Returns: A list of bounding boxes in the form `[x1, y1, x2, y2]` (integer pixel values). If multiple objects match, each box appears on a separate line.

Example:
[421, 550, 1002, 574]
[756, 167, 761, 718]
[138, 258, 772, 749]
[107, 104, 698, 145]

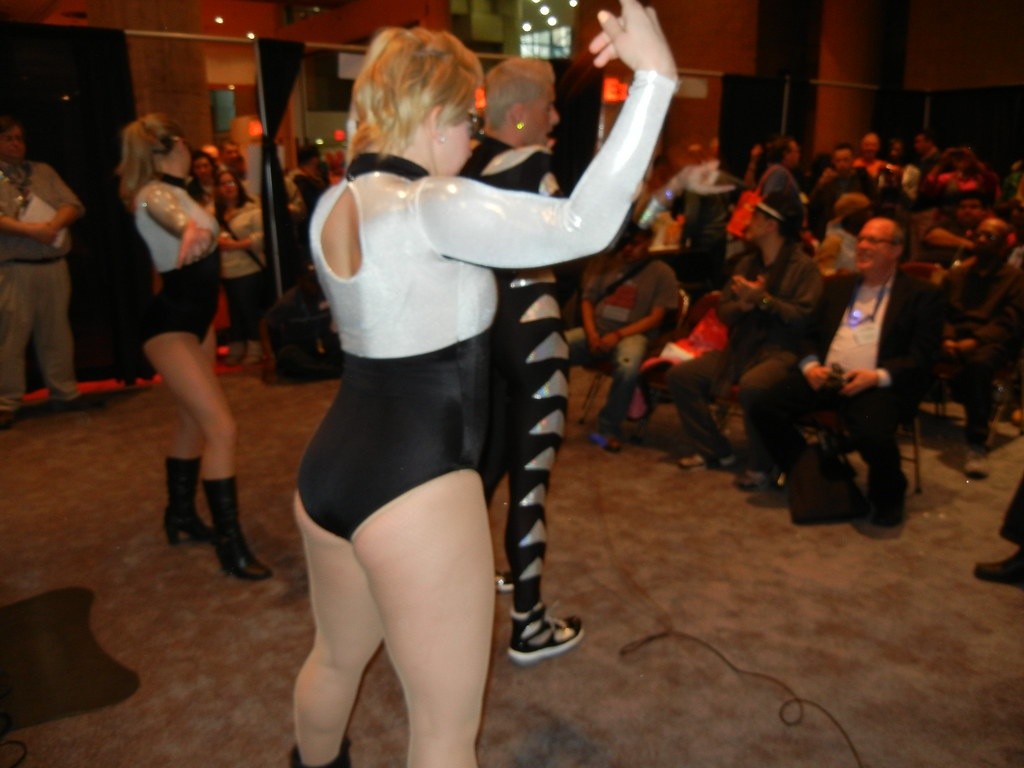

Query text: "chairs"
[565, 213, 1024, 495]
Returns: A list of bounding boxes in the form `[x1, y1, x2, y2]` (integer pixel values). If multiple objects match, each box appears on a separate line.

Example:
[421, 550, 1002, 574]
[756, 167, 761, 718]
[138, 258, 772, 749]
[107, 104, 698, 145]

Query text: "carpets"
[0, 585, 142, 742]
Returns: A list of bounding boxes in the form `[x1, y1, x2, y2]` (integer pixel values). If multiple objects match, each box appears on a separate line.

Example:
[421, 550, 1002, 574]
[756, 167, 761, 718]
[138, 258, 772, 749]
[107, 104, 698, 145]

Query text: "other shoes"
[680, 455, 713, 468]
[225, 341, 265, 365]
[965, 449, 994, 476]
[597, 433, 621, 454]
[872, 508, 903, 528]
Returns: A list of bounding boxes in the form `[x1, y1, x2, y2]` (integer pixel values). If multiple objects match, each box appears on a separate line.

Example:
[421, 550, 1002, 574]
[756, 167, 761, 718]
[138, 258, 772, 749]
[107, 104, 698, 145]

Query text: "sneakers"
[508, 615, 585, 665]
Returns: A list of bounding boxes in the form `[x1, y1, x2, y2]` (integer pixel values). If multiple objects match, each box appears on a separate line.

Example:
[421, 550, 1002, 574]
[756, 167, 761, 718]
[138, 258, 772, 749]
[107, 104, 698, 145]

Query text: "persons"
[463, 56, 584, 663]
[285, 144, 340, 356]
[567, 132, 1024, 586]
[191, 142, 263, 364]
[287, 0, 679, 768]
[120, 112, 273, 581]
[0, 119, 99, 427]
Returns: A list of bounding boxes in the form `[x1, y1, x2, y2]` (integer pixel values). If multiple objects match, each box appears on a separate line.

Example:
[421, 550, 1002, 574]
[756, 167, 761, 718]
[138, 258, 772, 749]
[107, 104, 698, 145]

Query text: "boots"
[163, 456, 212, 546]
[203, 477, 272, 580]
[493, 571, 514, 592]
[291, 738, 352, 768]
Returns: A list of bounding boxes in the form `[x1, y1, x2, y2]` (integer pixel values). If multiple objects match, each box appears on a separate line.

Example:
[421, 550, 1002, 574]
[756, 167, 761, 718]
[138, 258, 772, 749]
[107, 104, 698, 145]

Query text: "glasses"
[857, 234, 896, 247]
[465, 113, 486, 135]
[1, 135, 24, 142]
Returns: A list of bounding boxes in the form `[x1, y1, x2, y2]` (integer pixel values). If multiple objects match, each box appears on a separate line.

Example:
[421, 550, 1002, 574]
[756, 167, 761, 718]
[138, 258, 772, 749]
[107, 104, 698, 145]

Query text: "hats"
[750, 189, 805, 230]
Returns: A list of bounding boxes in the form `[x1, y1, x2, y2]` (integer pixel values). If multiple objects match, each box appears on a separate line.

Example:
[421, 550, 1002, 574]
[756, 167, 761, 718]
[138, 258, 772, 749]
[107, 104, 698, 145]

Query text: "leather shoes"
[974, 549, 1024, 587]
[2, 392, 104, 428]
